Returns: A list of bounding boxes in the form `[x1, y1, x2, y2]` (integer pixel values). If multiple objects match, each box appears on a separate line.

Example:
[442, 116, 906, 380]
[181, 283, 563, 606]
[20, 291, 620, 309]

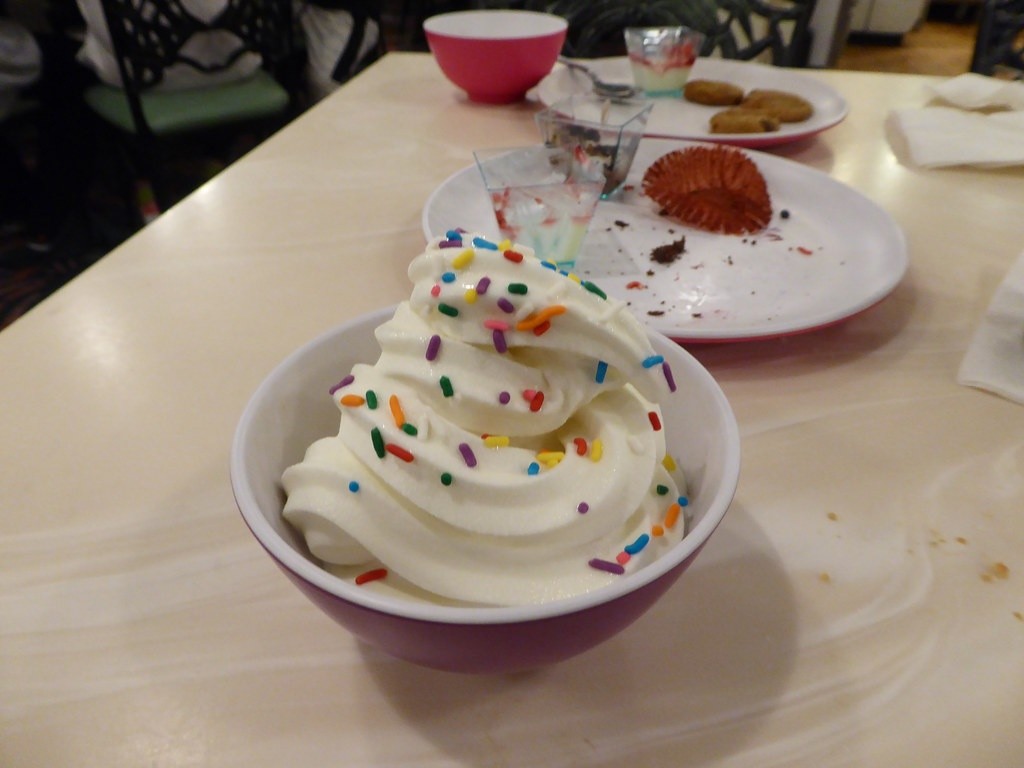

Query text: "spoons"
[558, 54, 635, 96]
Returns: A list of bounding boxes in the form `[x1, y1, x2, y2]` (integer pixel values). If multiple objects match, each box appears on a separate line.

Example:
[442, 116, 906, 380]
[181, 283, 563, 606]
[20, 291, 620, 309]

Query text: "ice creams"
[279, 227, 687, 609]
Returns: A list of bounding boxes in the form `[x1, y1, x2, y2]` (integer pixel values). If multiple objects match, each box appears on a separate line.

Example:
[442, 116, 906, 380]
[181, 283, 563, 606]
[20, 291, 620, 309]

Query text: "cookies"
[739, 89, 814, 123]
[683, 80, 743, 106]
[710, 108, 780, 133]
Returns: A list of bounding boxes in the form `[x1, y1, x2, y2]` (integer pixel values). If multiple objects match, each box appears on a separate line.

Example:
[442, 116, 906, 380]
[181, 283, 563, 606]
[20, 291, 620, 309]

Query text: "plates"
[422, 137, 911, 343]
[525, 54, 852, 150]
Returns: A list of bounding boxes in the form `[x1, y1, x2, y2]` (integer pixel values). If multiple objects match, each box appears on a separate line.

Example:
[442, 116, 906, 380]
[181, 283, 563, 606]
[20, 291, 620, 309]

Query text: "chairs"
[45, 0, 819, 218]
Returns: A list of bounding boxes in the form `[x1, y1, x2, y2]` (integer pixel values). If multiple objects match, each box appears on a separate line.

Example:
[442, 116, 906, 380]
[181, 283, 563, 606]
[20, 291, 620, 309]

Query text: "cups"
[625, 24, 708, 97]
[536, 92, 654, 199]
[472, 144, 611, 270]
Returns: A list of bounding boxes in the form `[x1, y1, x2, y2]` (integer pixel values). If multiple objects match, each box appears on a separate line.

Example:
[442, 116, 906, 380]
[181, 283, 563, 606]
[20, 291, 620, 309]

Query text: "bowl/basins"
[422, 10, 570, 105]
[230, 305, 744, 674]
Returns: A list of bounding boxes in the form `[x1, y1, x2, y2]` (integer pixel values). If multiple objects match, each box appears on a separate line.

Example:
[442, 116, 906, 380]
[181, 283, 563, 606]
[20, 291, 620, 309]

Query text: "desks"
[0, 51, 1024, 768]
